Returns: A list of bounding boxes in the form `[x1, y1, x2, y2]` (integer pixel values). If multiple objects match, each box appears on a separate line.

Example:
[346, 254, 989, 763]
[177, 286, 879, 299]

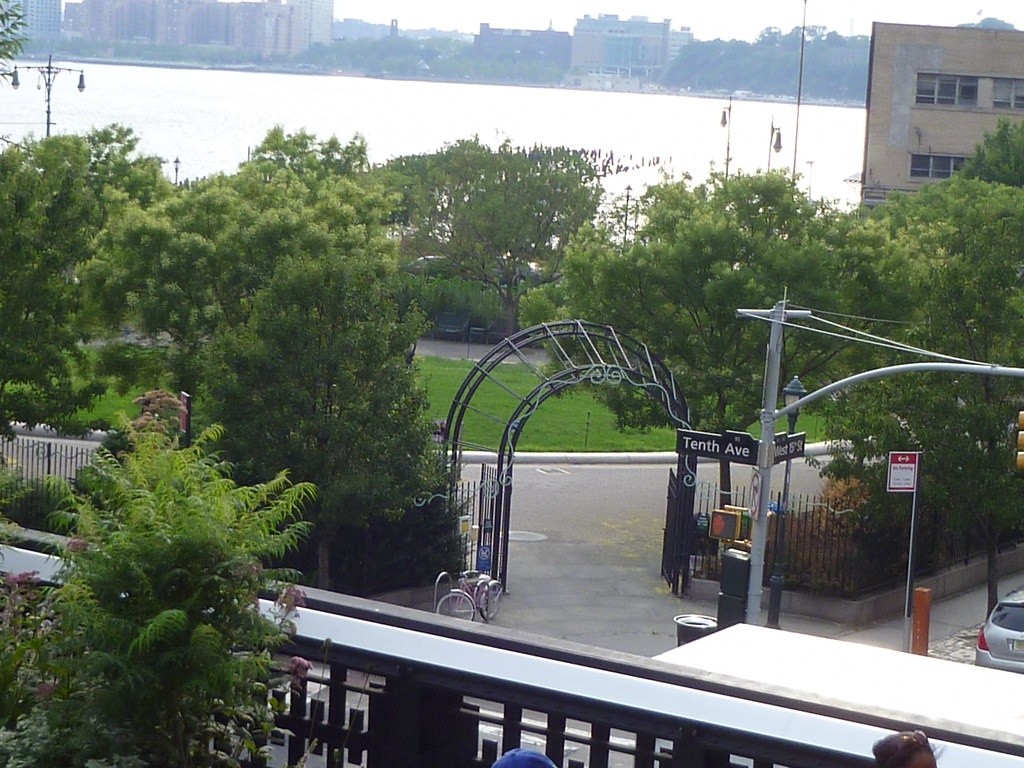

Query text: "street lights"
[764, 376, 810, 638]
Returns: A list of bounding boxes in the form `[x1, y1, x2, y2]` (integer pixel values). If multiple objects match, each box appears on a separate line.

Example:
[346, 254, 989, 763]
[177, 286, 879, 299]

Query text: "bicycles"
[436, 570, 503, 622]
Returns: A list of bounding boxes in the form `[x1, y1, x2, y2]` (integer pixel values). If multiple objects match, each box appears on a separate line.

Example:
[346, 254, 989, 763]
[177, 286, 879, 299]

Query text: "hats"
[491, 748, 557, 768]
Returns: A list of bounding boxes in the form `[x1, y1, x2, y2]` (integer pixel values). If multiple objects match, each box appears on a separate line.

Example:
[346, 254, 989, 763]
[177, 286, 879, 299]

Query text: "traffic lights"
[709, 510, 742, 542]
[1016, 411, 1024, 470]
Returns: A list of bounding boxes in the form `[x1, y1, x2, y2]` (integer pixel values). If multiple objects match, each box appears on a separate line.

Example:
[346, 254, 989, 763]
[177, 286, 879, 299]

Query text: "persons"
[871, 730, 938, 768]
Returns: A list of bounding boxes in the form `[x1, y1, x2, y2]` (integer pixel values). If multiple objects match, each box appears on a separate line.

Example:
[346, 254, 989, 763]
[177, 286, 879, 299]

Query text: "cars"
[973, 590, 1024, 674]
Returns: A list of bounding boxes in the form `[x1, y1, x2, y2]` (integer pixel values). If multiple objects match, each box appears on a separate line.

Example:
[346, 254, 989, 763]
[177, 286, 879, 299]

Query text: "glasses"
[884, 729, 927, 768]
